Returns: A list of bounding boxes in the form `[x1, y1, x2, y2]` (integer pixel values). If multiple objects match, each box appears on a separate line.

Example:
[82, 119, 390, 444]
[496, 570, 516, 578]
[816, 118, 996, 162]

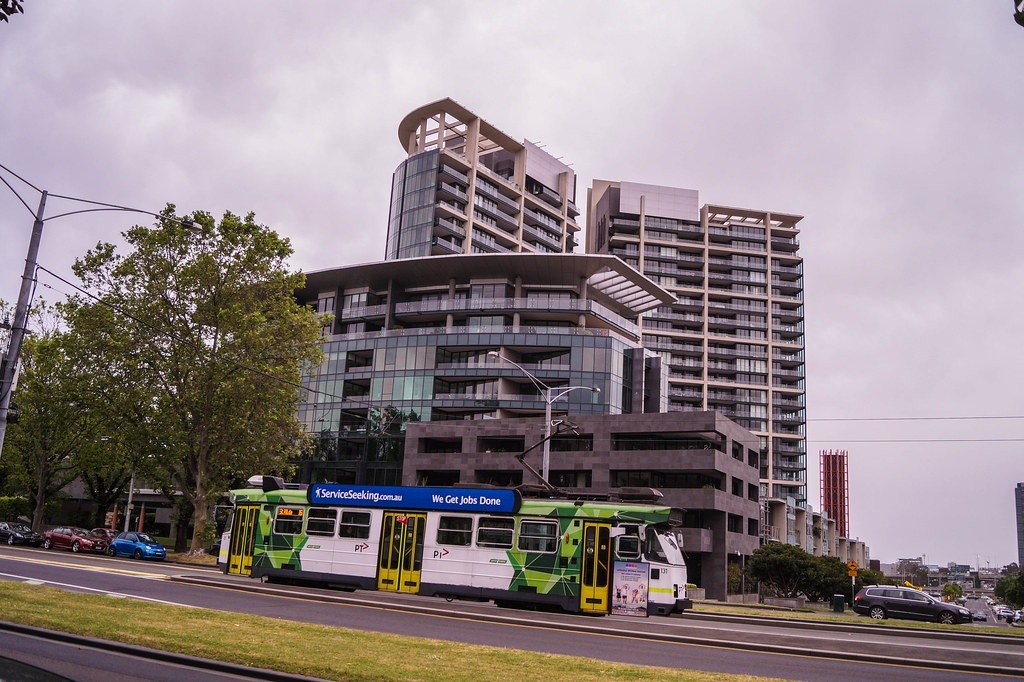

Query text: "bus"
[213, 475, 692, 618]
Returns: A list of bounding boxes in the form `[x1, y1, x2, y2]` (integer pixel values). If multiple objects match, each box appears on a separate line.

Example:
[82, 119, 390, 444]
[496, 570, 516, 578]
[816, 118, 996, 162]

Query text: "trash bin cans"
[833, 594, 845, 613]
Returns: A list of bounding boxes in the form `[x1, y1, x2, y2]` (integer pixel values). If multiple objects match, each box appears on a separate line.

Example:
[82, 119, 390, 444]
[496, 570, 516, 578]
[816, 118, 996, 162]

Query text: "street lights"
[0, 165, 203, 456]
[488, 351, 601, 487]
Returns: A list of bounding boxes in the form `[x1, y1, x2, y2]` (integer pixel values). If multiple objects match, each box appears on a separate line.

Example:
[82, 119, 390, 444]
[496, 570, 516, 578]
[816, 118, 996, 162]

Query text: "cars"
[91, 528, 122, 544]
[0, 522, 43, 547]
[43, 526, 107, 553]
[987, 598, 995, 605]
[108, 531, 167, 560]
[992, 605, 1024, 620]
[972, 611, 988, 622]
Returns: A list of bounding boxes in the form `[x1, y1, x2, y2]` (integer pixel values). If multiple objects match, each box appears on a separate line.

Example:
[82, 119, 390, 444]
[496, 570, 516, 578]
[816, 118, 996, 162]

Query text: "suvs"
[853, 584, 972, 625]
